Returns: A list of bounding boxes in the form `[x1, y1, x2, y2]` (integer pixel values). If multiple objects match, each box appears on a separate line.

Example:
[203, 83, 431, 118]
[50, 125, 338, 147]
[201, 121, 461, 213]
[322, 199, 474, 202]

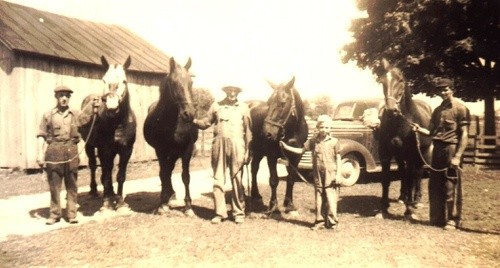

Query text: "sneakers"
[46, 217, 60, 225]
[235, 215, 245, 223]
[210, 216, 222, 224]
[68, 219, 78, 223]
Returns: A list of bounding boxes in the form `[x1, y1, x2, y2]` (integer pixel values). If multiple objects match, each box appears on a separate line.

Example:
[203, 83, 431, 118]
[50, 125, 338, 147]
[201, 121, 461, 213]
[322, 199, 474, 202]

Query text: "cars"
[276, 96, 436, 187]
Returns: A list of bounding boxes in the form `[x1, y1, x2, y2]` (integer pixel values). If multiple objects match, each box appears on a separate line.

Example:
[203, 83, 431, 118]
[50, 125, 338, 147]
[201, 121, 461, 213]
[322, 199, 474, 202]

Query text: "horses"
[78, 53, 138, 214]
[368, 57, 432, 220]
[142, 56, 196, 218]
[240, 76, 309, 220]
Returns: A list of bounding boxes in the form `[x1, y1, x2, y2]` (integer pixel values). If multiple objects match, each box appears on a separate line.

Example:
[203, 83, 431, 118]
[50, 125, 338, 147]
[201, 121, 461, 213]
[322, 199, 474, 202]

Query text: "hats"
[434, 78, 452, 88]
[222, 84, 242, 93]
[54, 86, 73, 93]
[316, 115, 334, 129]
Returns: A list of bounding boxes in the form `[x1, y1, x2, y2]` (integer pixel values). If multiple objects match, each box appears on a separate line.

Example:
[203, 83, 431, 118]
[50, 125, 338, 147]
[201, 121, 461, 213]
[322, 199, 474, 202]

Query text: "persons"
[408, 78, 470, 230]
[278, 114, 342, 230]
[35, 86, 81, 224]
[190, 81, 250, 224]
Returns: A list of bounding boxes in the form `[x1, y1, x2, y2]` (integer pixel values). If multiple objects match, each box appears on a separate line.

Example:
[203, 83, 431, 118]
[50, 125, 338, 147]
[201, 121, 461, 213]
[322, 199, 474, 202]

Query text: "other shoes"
[312, 222, 336, 232]
[430, 220, 458, 231]
